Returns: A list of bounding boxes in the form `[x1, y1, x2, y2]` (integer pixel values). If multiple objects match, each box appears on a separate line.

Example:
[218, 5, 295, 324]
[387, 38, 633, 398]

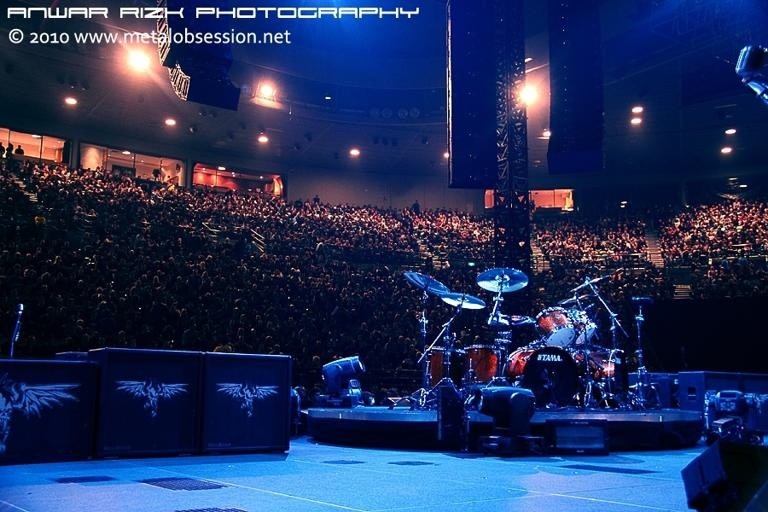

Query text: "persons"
[1, 139, 766, 405]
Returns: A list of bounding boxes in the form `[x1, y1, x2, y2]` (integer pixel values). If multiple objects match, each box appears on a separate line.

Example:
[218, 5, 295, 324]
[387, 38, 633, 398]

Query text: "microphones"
[17, 303, 24, 311]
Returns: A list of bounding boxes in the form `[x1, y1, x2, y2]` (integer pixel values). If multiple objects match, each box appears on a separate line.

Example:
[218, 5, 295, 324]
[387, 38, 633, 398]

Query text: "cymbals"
[401, 271, 451, 296]
[476, 267, 528, 292]
[557, 295, 591, 305]
[571, 275, 608, 292]
[440, 293, 486, 310]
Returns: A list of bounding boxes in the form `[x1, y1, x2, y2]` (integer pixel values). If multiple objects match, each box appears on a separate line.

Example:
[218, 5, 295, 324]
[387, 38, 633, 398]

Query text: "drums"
[505, 345, 578, 406]
[495, 331, 512, 344]
[462, 346, 508, 384]
[575, 352, 617, 386]
[567, 310, 597, 345]
[536, 307, 575, 349]
[428, 346, 466, 388]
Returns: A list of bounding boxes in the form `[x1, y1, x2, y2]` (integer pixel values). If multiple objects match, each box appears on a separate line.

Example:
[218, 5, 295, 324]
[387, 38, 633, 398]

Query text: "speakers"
[201, 351, 292, 452]
[0, 358, 97, 465]
[628, 371, 767, 433]
[680, 438, 767, 512]
[88, 349, 198, 458]
[156, 0, 240, 112]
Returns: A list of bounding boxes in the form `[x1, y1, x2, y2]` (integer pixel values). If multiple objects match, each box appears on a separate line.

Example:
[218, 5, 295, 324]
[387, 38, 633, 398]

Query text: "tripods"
[386, 321, 663, 413]
